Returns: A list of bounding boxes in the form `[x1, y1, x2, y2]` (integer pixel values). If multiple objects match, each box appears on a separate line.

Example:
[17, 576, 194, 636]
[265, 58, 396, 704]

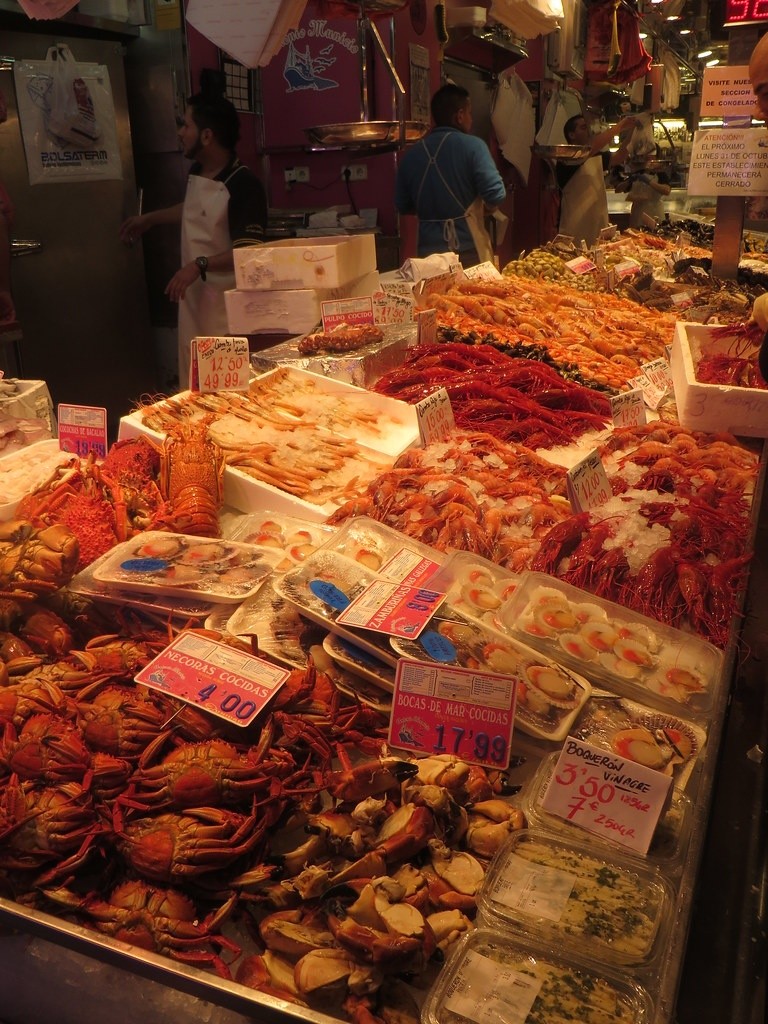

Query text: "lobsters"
[18, 414, 225, 569]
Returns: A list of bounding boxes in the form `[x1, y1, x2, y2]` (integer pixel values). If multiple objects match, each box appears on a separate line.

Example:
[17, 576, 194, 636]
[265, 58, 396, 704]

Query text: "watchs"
[195, 256, 208, 282]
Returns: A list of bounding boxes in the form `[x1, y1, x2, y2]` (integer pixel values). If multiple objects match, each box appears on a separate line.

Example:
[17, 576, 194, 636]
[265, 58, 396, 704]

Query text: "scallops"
[64, 506, 726, 800]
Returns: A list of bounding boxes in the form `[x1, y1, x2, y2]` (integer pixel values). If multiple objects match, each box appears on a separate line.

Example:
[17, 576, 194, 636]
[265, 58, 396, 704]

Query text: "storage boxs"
[669, 321, 768, 438]
[0, 379, 55, 420]
[248, 364, 421, 457]
[232, 233, 377, 292]
[117, 385, 396, 523]
[225, 270, 380, 334]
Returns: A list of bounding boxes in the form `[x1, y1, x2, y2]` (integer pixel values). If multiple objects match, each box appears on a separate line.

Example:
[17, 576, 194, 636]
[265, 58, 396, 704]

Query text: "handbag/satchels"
[13, 43, 126, 186]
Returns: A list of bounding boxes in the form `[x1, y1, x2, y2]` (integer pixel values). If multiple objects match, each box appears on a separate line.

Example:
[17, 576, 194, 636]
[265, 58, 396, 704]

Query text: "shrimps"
[128, 374, 402, 510]
[326, 278, 762, 651]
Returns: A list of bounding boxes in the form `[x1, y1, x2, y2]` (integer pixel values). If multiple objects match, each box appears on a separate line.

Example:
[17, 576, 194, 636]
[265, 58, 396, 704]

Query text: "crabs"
[0, 516, 391, 982]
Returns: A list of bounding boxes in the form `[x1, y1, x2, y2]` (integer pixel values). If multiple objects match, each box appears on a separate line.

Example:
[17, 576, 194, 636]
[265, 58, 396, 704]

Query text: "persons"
[394, 85, 507, 271]
[120, 91, 266, 395]
[615, 143, 673, 229]
[552, 112, 640, 246]
[746, 28, 768, 385]
